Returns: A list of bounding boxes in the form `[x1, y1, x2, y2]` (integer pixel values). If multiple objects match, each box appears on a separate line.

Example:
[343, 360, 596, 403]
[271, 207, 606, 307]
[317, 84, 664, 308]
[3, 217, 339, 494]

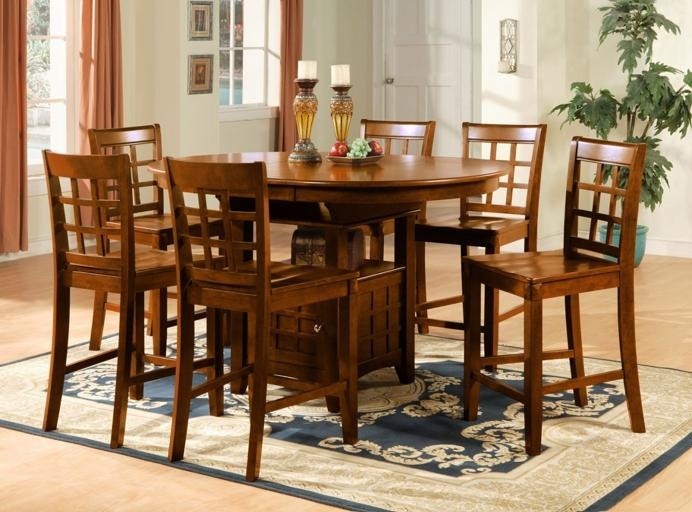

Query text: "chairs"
[298, 118, 436, 334]
[414, 122, 547, 373]
[462, 135, 647, 455]
[41, 149, 225, 449]
[163, 156, 360, 482]
[88, 123, 230, 365]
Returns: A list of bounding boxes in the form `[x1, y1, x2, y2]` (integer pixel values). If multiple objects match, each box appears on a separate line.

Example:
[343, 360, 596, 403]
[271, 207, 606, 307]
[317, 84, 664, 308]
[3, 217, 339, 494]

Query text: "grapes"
[346, 138, 372, 158]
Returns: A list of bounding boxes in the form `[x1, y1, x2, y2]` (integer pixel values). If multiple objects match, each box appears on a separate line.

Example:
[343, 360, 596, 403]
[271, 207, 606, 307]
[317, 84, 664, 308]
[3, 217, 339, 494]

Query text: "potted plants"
[547, 1, 692, 267]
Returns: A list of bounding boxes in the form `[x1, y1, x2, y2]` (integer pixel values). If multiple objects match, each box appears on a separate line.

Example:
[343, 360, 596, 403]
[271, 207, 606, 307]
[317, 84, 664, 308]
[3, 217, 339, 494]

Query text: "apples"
[368, 141, 383, 156]
[330, 142, 348, 157]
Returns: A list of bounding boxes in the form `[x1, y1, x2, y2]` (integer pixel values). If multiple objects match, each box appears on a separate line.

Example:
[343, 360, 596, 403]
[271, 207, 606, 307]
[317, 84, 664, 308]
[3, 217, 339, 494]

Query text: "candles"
[297, 60, 317, 79]
[331, 64, 351, 84]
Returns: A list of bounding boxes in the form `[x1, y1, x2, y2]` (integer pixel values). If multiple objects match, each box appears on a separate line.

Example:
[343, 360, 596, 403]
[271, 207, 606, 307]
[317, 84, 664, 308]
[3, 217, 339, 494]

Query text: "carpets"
[1, 306, 692, 511]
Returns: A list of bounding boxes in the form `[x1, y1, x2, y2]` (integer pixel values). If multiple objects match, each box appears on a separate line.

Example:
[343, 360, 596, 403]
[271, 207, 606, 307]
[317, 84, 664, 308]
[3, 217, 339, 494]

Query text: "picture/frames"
[187, 0, 213, 41]
[188, 55, 213, 94]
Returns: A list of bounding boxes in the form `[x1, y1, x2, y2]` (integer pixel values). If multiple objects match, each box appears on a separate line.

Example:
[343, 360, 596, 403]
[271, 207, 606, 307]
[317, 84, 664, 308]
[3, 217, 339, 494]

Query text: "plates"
[326, 154, 384, 167]
